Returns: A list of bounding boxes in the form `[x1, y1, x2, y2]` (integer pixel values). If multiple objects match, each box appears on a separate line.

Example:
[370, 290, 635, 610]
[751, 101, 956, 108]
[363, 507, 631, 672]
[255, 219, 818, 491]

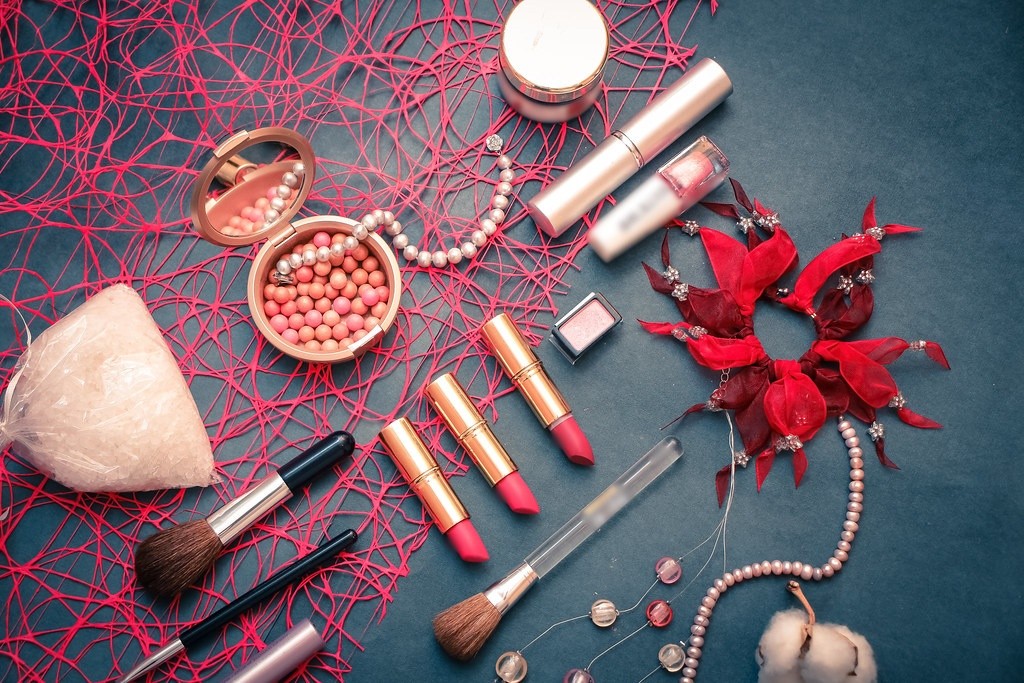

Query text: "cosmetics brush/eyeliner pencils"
[113, 528, 357, 681]
[430, 437, 685, 665]
[132, 431, 357, 607]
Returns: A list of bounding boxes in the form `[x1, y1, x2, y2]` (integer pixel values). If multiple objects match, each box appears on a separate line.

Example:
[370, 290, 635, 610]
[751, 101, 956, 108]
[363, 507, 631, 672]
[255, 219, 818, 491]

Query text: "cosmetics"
[493, 0, 611, 127]
[527, 59, 733, 238]
[377, 416, 492, 564]
[421, 369, 540, 516]
[480, 311, 596, 468]
[586, 134, 731, 263]
[550, 288, 624, 367]
[222, 618, 327, 683]
